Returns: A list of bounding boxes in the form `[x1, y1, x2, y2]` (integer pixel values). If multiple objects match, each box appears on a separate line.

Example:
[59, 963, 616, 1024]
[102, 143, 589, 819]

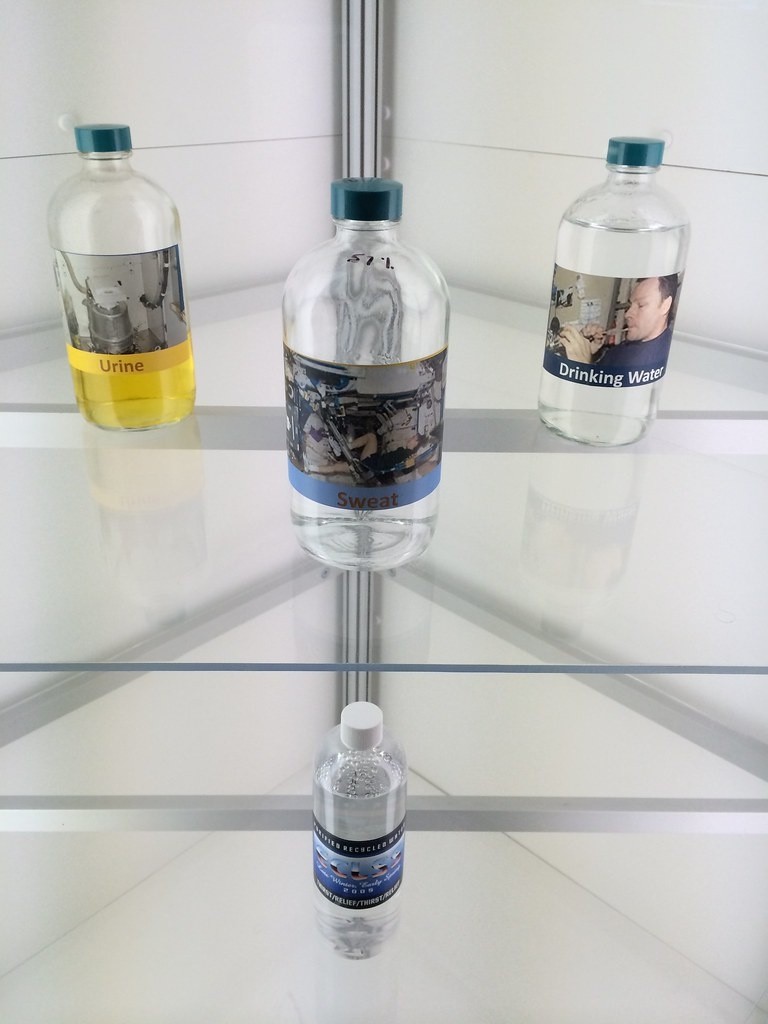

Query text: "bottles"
[47, 124, 195, 430]
[283, 177, 449, 571]
[312, 702, 406, 928]
[538, 136, 689, 447]
